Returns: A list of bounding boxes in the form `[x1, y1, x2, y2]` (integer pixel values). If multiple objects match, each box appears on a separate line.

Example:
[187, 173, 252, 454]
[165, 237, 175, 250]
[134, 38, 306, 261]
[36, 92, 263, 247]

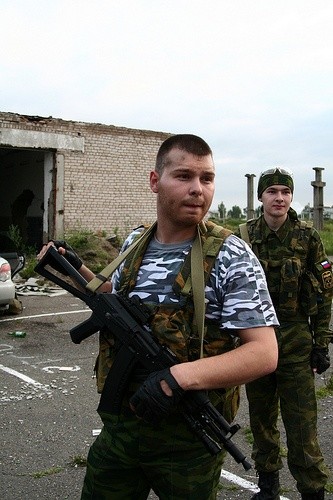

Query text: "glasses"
[261, 168, 290, 178]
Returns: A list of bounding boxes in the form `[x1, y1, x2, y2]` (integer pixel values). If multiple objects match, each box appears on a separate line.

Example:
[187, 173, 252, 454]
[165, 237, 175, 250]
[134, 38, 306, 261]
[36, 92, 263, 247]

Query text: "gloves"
[310, 343, 331, 375]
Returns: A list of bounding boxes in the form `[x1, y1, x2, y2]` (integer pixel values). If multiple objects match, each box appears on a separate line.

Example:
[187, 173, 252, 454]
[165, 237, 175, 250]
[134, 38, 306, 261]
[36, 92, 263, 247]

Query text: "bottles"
[7, 331, 26, 338]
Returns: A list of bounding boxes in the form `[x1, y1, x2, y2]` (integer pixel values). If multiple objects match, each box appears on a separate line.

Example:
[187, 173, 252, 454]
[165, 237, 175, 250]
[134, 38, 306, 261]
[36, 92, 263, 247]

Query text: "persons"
[37, 134, 281, 500]
[233, 168, 333, 500]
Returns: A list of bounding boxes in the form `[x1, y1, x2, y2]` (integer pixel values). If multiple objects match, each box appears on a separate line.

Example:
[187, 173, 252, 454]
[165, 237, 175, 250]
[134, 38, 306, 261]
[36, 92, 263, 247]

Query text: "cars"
[0, 233, 26, 306]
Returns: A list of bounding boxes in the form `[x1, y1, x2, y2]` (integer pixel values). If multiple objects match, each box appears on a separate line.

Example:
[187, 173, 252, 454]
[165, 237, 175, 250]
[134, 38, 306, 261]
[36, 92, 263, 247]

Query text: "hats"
[258, 171, 293, 196]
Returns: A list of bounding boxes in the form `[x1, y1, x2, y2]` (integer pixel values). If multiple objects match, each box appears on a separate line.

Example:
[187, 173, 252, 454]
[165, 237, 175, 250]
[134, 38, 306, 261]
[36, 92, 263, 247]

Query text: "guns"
[34, 245, 252, 473]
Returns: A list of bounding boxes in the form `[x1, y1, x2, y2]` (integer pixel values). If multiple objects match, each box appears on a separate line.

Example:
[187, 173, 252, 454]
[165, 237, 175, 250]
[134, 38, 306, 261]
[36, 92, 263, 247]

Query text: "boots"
[251, 472, 279, 500]
